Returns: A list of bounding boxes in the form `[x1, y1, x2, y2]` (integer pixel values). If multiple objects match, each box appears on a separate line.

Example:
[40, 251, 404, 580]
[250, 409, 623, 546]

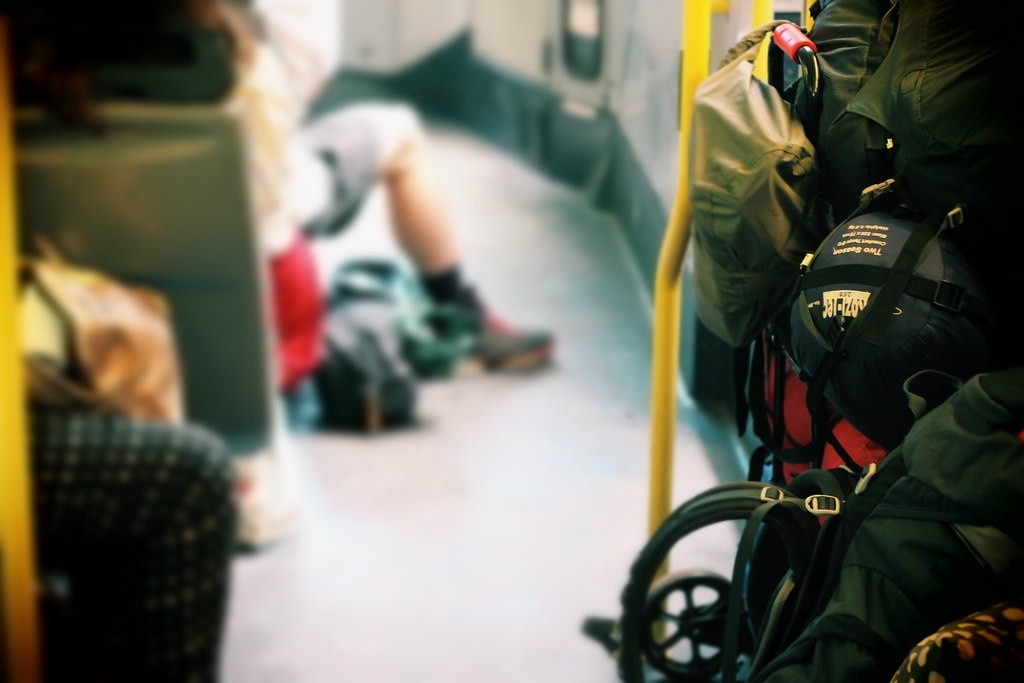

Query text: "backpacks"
[315, 297, 418, 432]
[693, 0, 1024, 683]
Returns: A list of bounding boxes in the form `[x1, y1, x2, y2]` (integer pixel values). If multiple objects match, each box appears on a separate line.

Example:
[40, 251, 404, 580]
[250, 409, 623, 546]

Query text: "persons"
[0, 1, 557, 683]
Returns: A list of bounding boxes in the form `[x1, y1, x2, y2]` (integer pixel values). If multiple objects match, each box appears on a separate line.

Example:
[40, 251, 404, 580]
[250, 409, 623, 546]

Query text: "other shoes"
[453, 283, 555, 375]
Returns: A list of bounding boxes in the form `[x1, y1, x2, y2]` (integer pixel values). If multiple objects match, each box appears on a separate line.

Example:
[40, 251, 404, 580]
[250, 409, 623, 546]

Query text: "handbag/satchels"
[330, 259, 468, 374]
